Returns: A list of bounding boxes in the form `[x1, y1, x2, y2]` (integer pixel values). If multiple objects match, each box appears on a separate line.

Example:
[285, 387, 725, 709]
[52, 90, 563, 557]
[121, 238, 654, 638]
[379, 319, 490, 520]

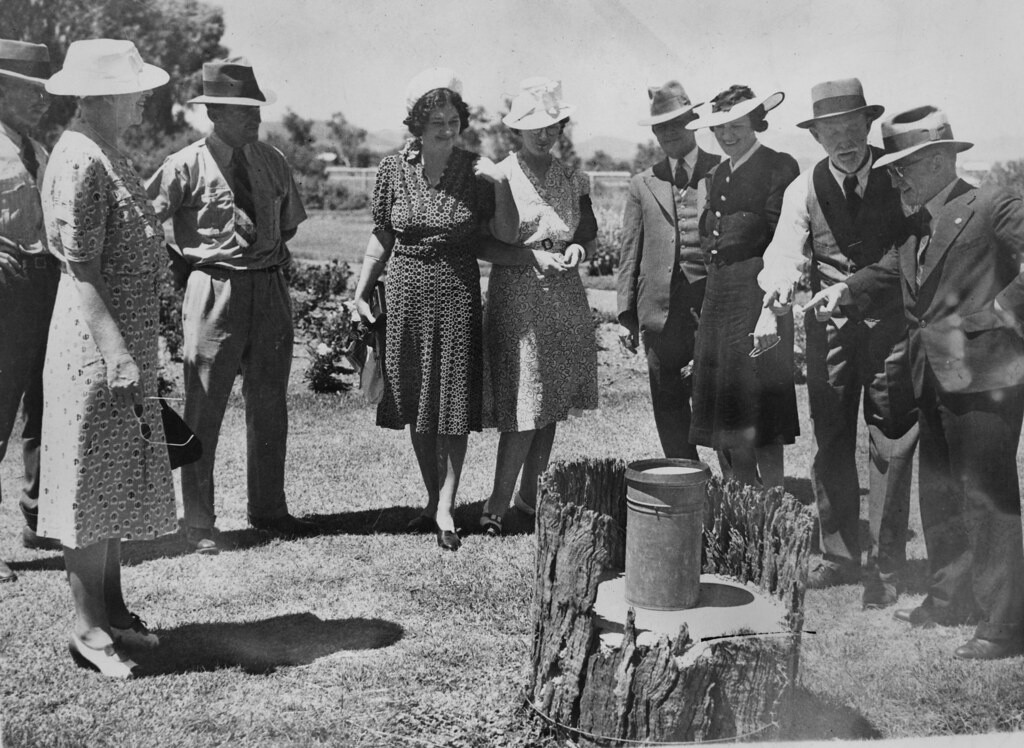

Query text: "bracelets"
[575, 242, 588, 263]
[365, 255, 386, 265]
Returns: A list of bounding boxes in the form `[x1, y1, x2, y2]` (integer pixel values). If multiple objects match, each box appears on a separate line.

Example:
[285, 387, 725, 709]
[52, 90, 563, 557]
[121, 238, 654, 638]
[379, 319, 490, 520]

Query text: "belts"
[18, 256, 58, 269]
[201, 264, 279, 273]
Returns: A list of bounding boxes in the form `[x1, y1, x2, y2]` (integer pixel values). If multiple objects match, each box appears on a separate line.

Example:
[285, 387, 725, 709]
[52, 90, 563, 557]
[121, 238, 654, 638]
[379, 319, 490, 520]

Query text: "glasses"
[531, 126, 560, 135]
[748, 332, 781, 357]
[131, 396, 194, 446]
[887, 153, 941, 176]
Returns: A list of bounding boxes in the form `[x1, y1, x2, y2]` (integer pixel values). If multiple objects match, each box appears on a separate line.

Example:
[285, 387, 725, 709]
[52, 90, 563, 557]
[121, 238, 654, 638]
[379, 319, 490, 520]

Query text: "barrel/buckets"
[625, 458, 712, 611]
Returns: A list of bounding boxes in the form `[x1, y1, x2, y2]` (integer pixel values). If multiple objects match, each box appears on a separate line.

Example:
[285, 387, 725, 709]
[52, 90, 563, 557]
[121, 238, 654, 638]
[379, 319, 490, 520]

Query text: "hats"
[502, 77, 575, 129]
[638, 80, 705, 125]
[45, 39, 170, 96]
[187, 56, 277, 106]
[685, 84, 785, 128]
[872, 105, 975, 169]
[0, 38, 52, 83]
[796, 78, 885, 128]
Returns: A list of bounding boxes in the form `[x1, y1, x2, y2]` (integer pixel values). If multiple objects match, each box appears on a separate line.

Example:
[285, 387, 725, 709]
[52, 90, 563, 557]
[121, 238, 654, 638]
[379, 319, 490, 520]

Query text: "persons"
[354, 68, 519, 550]
[685, 84, 801, 481]
[478, 79, 600, 535]
[616, 81, 723, 459]
[146, 63, 319, 554]
[43, 39, 173, 681]
[755, 77, 921, 611]
[802, 104, 1024, 660]
[0, 36, 63, 583]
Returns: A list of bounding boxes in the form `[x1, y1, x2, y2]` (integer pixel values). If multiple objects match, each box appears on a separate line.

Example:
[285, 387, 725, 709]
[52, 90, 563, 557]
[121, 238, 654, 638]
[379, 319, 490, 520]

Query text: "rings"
[132, 392, 140, 396]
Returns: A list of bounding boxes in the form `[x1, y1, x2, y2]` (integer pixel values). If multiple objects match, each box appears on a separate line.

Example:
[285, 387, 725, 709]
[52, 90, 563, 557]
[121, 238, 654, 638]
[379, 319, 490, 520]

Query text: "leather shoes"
[894, 607, 1013, 660]
[0, 511, 320, 581]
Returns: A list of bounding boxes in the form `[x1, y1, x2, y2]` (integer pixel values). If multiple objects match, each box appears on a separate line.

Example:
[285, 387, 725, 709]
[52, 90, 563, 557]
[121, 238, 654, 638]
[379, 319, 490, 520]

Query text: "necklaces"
[89, 126, 135, 165]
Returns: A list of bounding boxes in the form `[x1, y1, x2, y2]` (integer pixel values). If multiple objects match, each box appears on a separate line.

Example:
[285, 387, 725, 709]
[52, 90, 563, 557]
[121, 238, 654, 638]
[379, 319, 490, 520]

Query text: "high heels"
[67, 613, 170, 683]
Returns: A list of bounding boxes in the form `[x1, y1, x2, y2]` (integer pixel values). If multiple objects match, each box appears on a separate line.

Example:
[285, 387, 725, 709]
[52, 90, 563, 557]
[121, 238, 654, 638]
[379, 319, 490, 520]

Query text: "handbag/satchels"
[159, 396, 203, 469]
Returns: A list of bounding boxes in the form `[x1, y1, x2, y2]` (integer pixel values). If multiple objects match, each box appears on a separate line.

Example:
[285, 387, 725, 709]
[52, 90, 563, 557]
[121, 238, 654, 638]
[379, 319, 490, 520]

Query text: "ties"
[232, 148, 258, 254]
[675, 158, 688, 192]
[19, 131, 40, 176]
[844, 175, 860, 224]
[915, 206, 931, 295]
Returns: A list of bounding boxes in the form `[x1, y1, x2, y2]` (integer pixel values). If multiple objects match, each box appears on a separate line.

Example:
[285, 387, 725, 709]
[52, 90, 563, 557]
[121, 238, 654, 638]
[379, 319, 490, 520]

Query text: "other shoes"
[806, 562, 898, 609]
[406, 490, 536, 550]
